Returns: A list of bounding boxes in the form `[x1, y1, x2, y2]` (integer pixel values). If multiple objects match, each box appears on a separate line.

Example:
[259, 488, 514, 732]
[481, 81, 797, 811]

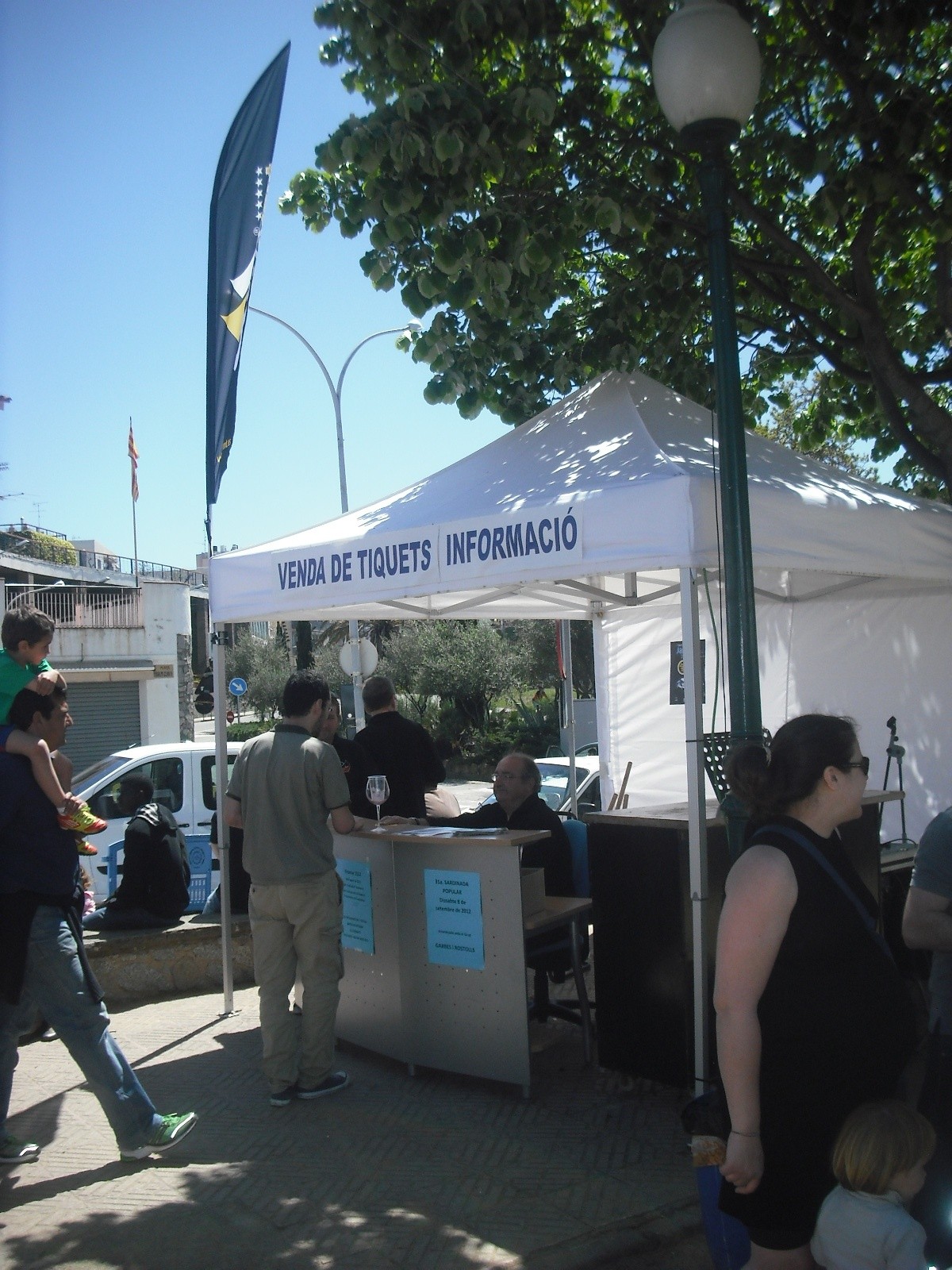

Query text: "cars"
[468, 743, 602, 824]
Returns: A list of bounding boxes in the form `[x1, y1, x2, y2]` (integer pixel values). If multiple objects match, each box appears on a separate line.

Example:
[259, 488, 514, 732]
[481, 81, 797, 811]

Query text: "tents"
[208, 363, 952, 1099]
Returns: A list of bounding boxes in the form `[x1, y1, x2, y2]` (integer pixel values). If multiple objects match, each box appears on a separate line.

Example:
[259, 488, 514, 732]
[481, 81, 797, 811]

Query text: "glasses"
[491, 772, 528, 782]
[829, 755, 871, 776]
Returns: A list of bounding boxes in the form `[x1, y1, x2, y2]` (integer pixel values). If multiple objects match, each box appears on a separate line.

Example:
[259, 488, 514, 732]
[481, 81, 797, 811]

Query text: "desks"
[580, 787, 909, 1084]
[514, 896, 597, 1057]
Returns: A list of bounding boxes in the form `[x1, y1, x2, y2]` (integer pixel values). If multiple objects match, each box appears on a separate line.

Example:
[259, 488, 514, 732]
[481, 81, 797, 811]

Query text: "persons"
[79, 773, 191, 930]
[713, 714, 952, 1270]
[226, 668, 364, 1106]
[424, 781, 461, 818]
[352, 675, 445, 819]
[0, 603, 108, 856]
[0, 687, 199, 1163]
[381, 753, 575, 968]
[317, 690, 353, 785]
[203, 810, 251, 914]
[80, 865, 95, 916]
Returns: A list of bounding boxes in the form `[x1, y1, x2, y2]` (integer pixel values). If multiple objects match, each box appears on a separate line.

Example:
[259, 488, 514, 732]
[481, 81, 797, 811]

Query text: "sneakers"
[59, 807, 109, 834]
[270, 1083, 297, 1105]
[73, 838, 98, 855]
[299, 1071, 348, 1098]
[3, 1136, 40, 1164]
[120, 1111, 198, 1162]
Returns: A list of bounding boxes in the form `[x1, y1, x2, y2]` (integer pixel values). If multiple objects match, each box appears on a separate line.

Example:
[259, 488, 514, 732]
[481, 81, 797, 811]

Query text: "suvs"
[69, 739, 243, 915]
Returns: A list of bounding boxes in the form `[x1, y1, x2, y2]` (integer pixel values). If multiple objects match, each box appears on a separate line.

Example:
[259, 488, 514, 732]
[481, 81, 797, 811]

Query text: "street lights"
[224, 288, 424, 735]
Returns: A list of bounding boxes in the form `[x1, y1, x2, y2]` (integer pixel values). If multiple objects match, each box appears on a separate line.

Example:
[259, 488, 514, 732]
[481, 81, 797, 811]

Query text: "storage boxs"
[519, 866, 549, 919]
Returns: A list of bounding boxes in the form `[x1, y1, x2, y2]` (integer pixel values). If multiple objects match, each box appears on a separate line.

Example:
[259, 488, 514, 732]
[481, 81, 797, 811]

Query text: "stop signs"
[225, 711, 235, 723]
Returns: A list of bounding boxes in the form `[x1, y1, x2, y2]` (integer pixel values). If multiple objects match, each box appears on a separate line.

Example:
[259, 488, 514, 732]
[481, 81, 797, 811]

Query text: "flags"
[128, 428, 139, 466]
[208, 39, 290, 507]
[132, 461, 139, 502]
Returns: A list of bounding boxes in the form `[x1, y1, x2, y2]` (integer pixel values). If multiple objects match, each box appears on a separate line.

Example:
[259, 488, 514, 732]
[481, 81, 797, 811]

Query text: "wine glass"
[366, 775, 390, 834]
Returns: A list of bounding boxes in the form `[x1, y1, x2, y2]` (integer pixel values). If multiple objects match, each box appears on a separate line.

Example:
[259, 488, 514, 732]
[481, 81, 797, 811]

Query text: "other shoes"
[40, 1023, 60, 1040]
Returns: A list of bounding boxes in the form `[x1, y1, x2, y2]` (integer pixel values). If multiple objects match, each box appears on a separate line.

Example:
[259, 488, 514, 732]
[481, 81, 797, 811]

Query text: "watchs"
[408, 817, 419, 826]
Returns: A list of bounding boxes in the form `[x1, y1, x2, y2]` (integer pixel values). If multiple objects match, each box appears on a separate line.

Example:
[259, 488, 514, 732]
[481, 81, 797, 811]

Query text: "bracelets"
[731, 1131, 761, 1137]
